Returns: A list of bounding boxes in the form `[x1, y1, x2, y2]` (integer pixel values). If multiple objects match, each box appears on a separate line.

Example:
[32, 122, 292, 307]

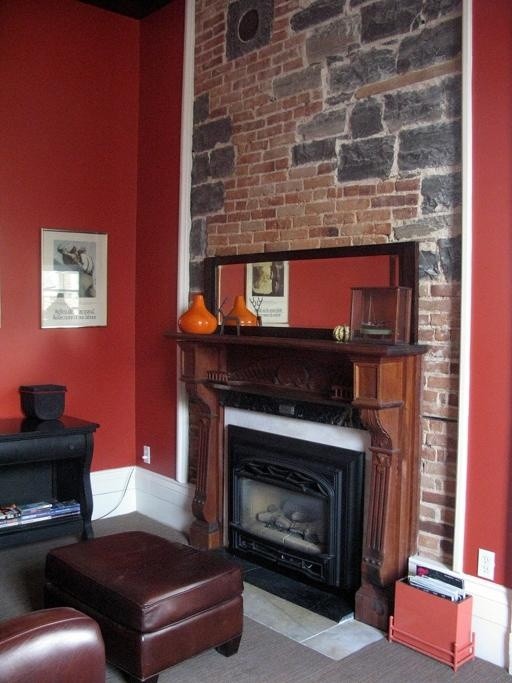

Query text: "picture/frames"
[40, 227, 109, 330]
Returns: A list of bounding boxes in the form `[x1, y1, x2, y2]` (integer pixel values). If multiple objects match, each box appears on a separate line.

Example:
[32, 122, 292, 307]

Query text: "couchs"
[0, 607, 107, 683]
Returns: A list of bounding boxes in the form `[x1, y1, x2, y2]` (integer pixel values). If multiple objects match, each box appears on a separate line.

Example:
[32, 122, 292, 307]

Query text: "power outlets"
[477, 548, 496, 581]
[143, 446, 150, 465]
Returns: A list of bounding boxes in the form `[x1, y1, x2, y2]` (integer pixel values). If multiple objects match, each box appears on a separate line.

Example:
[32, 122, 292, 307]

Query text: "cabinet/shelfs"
[0, 415, 100, 550]
[160, 241, 429, 630]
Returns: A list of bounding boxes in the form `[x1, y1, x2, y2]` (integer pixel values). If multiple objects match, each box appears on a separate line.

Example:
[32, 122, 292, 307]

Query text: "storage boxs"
[389, 576, 476, 672]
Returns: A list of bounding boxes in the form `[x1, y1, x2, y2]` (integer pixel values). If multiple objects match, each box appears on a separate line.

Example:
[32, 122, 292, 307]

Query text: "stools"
[42, 530, 244, 683]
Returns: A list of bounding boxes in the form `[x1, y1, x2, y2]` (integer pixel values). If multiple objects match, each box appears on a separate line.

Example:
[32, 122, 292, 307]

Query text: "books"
[406, 555, 467, 602]
[0, 497, 81, 529]
